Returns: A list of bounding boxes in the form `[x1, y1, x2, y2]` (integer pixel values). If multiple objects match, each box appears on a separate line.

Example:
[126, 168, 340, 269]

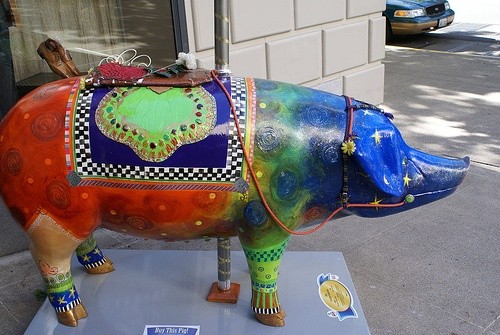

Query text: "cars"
[383, 0, 455, 35]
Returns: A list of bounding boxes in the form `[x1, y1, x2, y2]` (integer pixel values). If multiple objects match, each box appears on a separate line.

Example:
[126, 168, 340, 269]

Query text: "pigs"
[0, 74, 471, 328]
